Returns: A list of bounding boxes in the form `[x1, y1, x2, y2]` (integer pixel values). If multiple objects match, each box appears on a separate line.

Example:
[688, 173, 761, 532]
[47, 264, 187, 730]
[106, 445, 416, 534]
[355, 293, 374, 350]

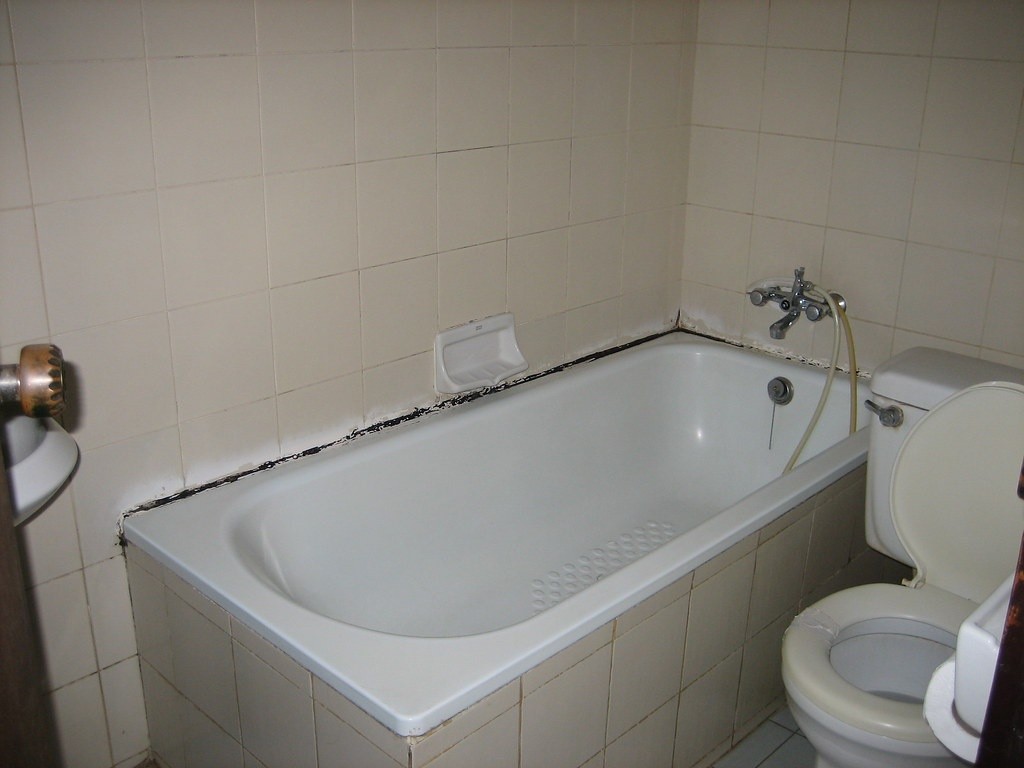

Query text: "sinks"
[2, 410, 79, 528]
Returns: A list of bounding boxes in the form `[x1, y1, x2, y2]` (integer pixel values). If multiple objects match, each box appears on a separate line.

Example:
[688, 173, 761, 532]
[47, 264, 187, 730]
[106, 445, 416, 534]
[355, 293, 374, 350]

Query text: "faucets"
[749, 267, 846, 340]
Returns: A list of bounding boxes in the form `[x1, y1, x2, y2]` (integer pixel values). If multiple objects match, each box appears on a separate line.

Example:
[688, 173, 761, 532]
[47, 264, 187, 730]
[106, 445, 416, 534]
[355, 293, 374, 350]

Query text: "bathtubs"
[124, 330, 900, 768]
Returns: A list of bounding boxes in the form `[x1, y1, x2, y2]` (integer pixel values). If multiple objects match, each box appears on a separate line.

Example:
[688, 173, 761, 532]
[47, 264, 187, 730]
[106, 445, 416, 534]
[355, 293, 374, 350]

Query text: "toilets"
[780, 344, 1024, 767]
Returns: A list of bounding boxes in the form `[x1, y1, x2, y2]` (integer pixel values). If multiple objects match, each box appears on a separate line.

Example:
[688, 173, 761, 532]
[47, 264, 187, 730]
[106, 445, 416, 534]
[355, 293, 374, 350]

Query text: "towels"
[971, 468, 1024, 767]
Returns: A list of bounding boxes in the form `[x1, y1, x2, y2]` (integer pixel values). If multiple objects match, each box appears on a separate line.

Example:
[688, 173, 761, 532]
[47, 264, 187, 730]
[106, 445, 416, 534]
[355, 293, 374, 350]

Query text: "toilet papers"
[923, 651, 981, 766]
